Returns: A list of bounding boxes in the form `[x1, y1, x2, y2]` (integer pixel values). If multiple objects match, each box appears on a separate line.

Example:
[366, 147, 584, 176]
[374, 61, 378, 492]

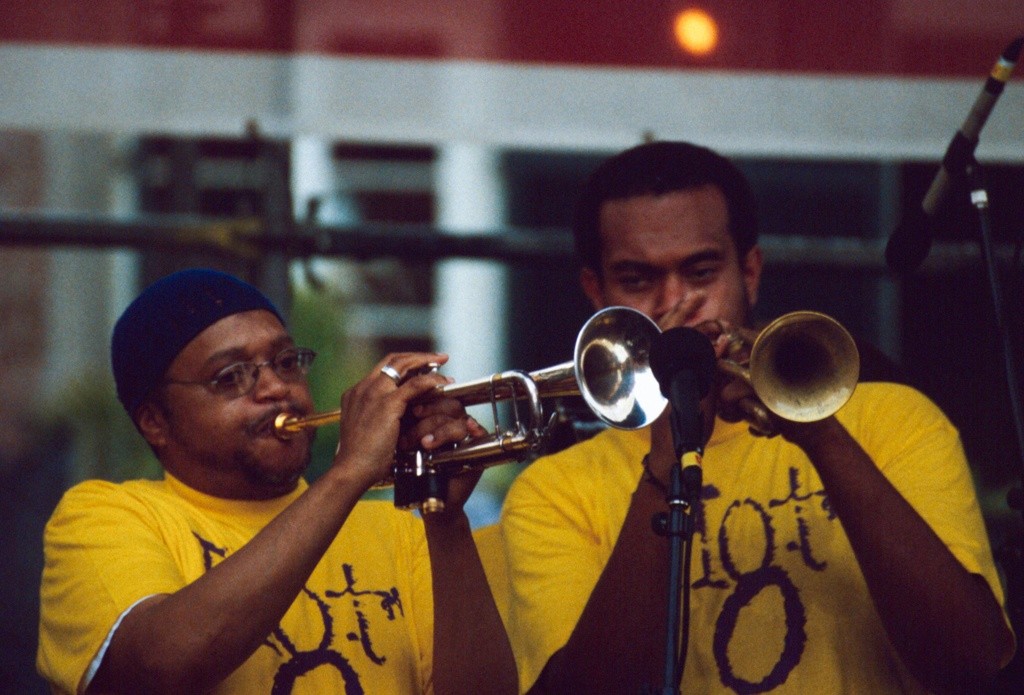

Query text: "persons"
[36, 264, 520, 695]
[499, 140, 1017, 695]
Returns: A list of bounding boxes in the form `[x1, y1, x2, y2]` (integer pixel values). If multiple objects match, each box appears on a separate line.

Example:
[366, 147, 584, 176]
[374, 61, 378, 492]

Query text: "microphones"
[884, 36, 1024, 277]
[649, 326, 719, 499]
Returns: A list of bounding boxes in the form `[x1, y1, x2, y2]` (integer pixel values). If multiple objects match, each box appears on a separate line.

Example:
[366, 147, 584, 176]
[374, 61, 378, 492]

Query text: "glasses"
[158, 348, 318, 397]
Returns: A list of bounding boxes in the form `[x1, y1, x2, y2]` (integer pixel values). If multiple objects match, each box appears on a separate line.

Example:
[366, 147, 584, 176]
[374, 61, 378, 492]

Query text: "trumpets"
[272, 307, 672, 515]
[712, 305, 862, 426]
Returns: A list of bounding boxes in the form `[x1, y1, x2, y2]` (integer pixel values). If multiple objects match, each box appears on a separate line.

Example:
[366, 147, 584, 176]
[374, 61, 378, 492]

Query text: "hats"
[111, 268, 281, 420]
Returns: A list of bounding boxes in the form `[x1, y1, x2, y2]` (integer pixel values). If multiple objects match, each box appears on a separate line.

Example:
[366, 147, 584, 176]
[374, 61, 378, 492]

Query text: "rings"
[380, 366, 401, 384]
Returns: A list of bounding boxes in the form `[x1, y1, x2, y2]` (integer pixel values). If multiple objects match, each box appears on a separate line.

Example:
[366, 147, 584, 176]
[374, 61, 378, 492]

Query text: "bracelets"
[642, 453, 671, 499]
